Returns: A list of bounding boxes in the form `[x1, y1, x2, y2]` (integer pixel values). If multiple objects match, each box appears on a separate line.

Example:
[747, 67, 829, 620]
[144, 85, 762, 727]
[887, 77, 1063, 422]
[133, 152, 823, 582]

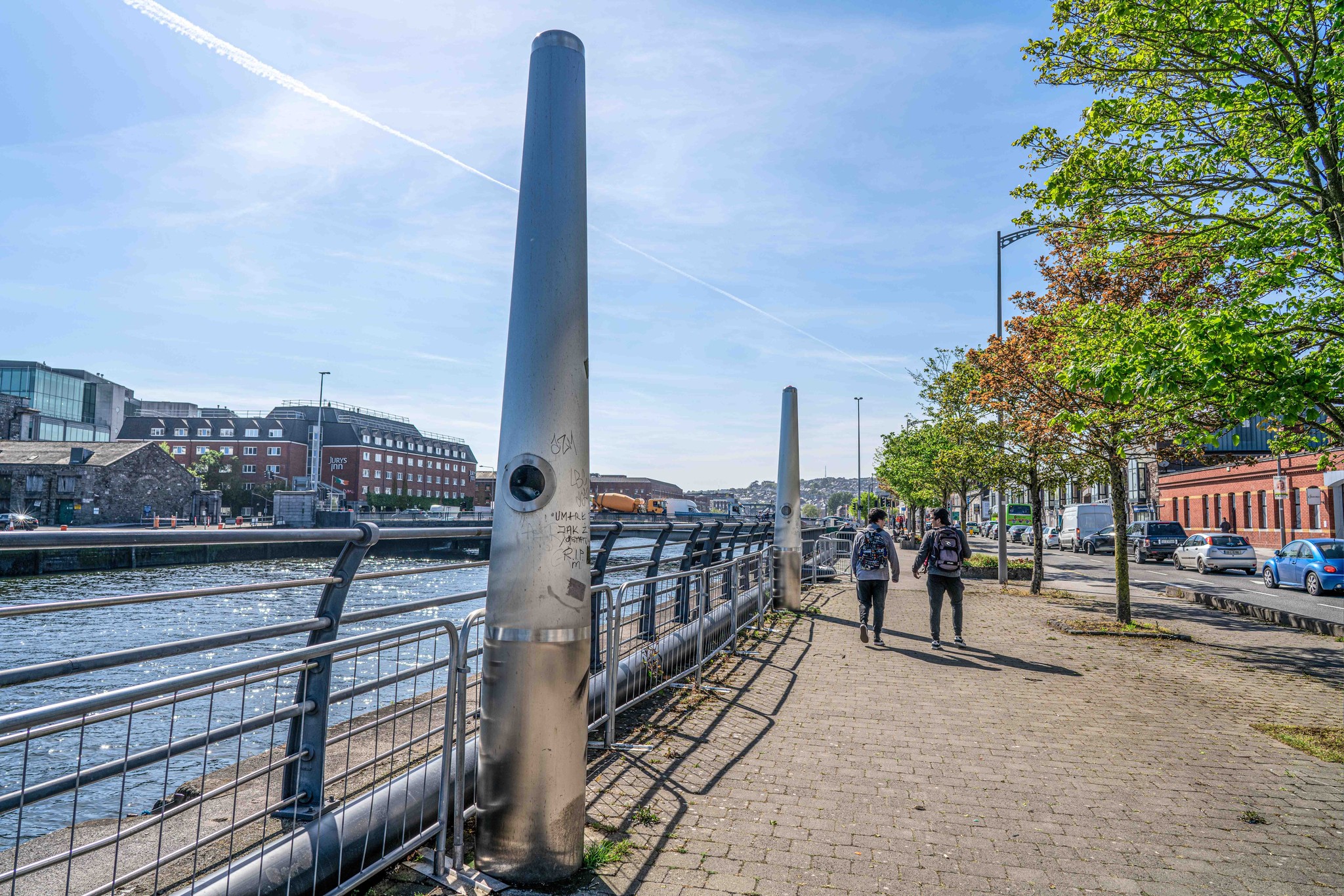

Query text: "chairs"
[1289, 550, 1304, 558]
[1324, 548, 1337, 558]
[1212, 539, 1217, 545]
[1306, 550, 1314, 558]
[1217, 538, 1229, 545]
[1231, 538, 1240, 546]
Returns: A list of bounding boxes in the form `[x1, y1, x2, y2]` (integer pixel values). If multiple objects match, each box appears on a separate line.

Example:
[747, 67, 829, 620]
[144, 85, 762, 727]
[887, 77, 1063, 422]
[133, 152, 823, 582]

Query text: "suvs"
[1126, 519, 1189, 564]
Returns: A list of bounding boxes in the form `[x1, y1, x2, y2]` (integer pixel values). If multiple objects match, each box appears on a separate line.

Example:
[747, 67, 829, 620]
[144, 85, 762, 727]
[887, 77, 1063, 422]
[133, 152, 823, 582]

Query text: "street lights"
[314, 371, 330, 492]
[854, 397, 864, 524]
[480, 465, 493, 512]
[869, 483, 880, 493]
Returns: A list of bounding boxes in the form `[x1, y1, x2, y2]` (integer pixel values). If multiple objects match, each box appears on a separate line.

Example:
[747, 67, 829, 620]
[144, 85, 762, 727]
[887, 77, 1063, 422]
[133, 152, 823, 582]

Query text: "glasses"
[930, 518, 934, 520]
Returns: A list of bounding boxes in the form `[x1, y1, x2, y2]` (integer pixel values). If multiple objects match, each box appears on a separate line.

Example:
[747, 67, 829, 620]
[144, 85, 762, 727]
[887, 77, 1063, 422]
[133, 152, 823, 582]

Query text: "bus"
[989, 503, 1033, 527]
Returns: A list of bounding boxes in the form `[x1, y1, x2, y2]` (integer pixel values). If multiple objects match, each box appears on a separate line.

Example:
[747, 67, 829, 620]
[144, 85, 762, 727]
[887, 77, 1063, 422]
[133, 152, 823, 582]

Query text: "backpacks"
[858, 528, 888, 570]
[932, 527, 963, 573]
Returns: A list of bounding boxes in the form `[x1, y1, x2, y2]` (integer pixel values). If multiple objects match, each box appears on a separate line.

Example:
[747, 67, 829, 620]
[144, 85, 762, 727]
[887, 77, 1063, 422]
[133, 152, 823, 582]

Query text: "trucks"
[430, 505, 461, 519]
[653, 498, 752, 524]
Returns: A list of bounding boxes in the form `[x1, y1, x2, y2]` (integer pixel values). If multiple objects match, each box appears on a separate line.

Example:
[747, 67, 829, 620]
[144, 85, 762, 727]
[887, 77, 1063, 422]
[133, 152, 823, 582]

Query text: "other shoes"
[860, 624, 869, 643]
[954, 636, 966, 647]
[873, 637, 884, 645]
[931, 641, 941, 649]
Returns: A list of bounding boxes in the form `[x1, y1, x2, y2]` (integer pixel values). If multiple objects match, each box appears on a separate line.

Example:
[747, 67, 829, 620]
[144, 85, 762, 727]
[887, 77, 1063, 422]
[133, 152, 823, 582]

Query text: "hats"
[930, 507, 952, 526]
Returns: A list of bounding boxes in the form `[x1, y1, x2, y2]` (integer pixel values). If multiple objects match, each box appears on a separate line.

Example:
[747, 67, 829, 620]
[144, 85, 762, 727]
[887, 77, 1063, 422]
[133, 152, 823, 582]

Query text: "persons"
[1219, 517, 1231, 533]
[733, 507, 739, 513]
[757, 511, 776, 525]
[662, 507, 668, 522]
[890, 515, 933, 531]
[852, 507, 900, 645]
[958, 520, 961, 526]
[690, 509, 695, 512]
[912, 507, 972, 649]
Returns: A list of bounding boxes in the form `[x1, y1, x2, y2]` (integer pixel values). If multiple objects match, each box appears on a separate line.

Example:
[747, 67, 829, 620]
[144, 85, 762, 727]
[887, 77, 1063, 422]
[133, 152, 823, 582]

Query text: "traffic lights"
[947, 502, 950, 511]
[264, 469, 268, 478]
[925, 514, 930, 518]
[339, 499, 343, 507]
[852, 502, 863, 511]
[880, 497, 890, 507]
[460, 501, 467, 509]
[324, 487, 329, 500]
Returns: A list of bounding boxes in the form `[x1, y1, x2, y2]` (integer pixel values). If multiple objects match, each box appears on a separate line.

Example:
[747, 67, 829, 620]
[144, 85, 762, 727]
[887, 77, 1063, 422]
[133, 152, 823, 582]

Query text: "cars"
[391, 509, 429, 520]
[1262, 538, 1344, 596]
[1082, 524, 1130, 555]
[815, 516, 860, 529]
[0, 513, 39, 531]
[954, 520, 1059, 550]
[1173, 532, 1258, 575]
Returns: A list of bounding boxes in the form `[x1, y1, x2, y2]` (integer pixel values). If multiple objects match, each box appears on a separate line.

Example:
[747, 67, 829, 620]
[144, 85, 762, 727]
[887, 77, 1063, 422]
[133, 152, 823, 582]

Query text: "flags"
[333, 477, 349, 485]
[344, 489, 354, 494]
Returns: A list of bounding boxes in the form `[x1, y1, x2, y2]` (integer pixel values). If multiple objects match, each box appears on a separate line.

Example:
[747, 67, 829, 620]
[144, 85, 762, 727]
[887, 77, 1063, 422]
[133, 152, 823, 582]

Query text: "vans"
[1056, 503, 1114, 553]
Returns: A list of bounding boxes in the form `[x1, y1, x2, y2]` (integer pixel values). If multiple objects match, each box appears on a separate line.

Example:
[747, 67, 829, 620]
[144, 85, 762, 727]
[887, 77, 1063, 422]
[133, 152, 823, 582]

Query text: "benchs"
[893, 529, 904, 543]
[899, 532, 915, 549]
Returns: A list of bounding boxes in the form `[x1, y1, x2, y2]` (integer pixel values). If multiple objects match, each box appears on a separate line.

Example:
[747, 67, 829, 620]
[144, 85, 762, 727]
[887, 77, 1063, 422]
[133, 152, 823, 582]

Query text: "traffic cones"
[9, 519, 14, 531]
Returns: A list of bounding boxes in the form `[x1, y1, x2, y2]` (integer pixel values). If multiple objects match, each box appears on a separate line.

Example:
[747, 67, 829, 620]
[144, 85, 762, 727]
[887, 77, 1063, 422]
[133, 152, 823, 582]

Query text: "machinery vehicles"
[589, 492, 668, 520]
